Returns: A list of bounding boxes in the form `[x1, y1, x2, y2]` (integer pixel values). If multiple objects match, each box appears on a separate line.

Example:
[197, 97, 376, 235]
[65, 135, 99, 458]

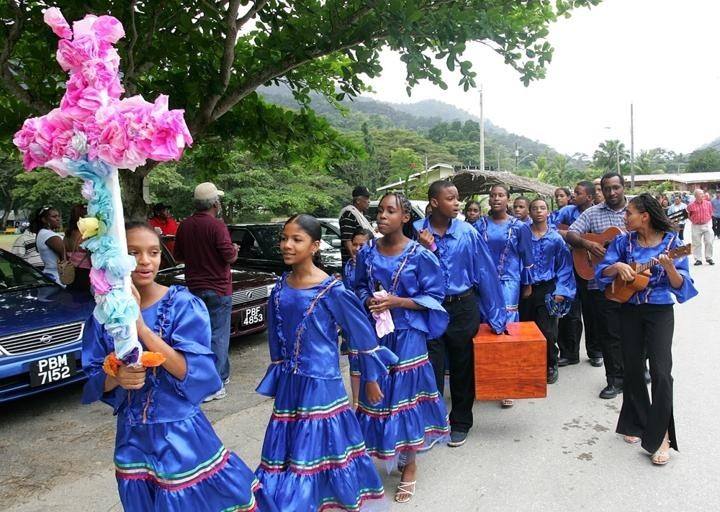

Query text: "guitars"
[555, 224, 573, 250]
[572, 208, 688, 281]
[604, 244, 691, 303]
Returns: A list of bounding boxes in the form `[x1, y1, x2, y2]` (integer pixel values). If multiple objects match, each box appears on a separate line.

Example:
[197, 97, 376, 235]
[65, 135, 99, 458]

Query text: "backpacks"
[56, 259, 75, 286]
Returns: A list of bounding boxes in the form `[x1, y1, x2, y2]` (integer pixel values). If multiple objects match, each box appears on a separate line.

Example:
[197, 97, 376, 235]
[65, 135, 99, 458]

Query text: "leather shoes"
[599, 384, 624, 400]
[590, 356, 605, 367]
[557, 355, 580, 367]
[546, 360, 559, 385]
[706, 259, 715, 266]
[693, 260, 702, 266]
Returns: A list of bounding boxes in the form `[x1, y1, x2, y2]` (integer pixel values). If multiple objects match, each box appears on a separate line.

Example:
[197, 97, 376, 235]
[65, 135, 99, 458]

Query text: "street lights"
[604, 126, 622, 178]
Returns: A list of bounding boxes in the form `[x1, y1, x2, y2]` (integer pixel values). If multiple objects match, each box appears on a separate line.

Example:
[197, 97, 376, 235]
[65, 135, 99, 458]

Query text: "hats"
[153, 203, 173, 213]
[352, 185, 374, 197]
[193, 181, 225, 202]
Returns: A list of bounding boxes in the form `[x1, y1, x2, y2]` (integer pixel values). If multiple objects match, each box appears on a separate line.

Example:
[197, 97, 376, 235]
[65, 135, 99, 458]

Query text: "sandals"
[649, 439, 671, 466]
[501, 399, 514, 407]
[623, 435, 642, 444]
[395, 472, 417, 503]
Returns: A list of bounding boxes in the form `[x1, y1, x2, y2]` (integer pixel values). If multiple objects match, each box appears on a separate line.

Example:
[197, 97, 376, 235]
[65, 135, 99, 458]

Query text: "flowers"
[12, 5, 192, 382]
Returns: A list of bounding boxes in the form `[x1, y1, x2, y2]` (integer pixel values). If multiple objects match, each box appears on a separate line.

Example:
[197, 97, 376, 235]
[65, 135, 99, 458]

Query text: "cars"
[0, 247, 100, 407]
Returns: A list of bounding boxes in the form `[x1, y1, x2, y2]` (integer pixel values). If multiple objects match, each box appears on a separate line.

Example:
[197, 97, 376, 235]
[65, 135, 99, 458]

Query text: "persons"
[251, 213, 399, 512]
[473, 182, 536, 408]
[63, 202, 91, 295]
[80, 219, 278, 512]
[424, 201, 433, 217]
[513, 196, 533, 226]
[518, 197, 577, 384]
[354, 192, 451, 504]
[464, 200, 482, 225]
[337, 228, 375, 410]
[339, 184, 375, 280]
[410, 179, 512, 446]
[149, 203, 179, 268]
[544, 172, 720, 466]
[28, 204, 65, 287]
[174, 182, 240, 403]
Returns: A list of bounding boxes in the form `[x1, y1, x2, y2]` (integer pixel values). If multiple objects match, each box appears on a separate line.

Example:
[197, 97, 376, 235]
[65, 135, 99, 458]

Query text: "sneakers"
[199, 386, 229, 404]
[224, 376, 230, 385]
[446, 430, 469, 448]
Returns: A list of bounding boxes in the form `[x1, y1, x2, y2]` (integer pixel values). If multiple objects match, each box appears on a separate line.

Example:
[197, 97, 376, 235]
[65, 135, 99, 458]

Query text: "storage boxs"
[469, 318, 549, 401]
